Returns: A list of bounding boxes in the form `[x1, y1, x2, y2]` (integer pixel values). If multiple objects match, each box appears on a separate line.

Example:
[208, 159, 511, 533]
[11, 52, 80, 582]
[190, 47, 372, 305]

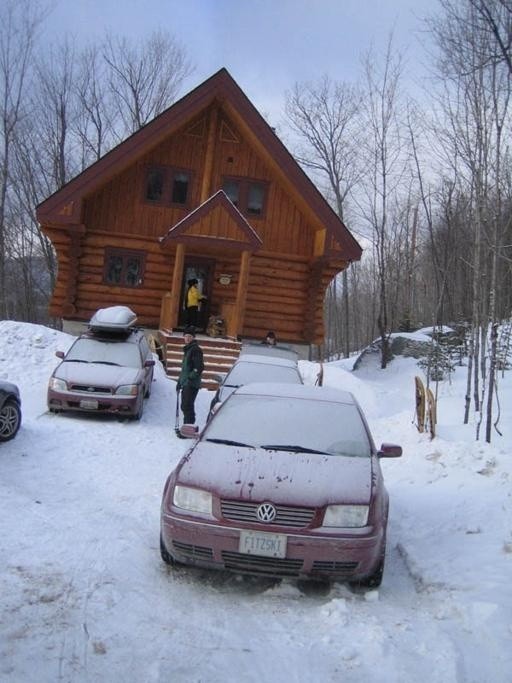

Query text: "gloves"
[176, 382, 181, 391]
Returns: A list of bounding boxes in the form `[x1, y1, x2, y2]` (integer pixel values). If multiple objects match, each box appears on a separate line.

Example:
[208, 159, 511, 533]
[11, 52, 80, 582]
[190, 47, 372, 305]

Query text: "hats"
[182, 326, 195, 336]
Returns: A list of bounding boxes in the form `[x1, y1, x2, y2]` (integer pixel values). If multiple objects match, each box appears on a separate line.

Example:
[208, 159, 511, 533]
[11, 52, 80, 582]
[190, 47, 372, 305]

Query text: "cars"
[44, 302, 160, 425]
[153, 381, 407, 593]
[208, 339, 308, 425]
[0, 375, 24, 443]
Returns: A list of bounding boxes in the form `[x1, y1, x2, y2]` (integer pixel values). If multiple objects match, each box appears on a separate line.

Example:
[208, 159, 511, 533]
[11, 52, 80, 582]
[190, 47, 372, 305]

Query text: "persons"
[186, 278, 208, 334]
[177, 326, 204, 425]
[260, 331, 278, 345]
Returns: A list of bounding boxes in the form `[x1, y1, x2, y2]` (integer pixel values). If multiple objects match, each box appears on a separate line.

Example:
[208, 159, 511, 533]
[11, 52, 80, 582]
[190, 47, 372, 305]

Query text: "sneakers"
[175, 430, 187, 438]
